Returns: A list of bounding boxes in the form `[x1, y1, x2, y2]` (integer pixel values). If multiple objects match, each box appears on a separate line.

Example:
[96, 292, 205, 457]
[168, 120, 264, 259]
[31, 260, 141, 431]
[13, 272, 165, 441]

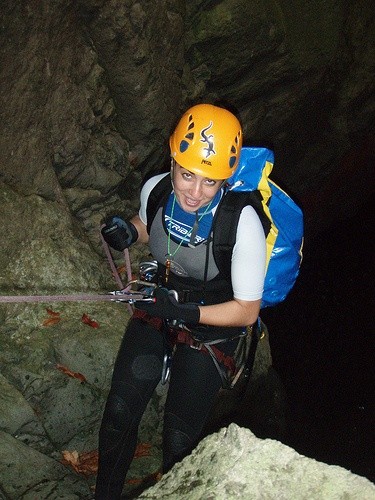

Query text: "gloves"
[101, 215, 138, 252]
[133, 288, 200, 324]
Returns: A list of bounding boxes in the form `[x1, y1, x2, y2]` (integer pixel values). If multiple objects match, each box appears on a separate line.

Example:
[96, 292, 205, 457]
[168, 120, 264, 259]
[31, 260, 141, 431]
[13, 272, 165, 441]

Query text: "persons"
[94, 103, 267, 500]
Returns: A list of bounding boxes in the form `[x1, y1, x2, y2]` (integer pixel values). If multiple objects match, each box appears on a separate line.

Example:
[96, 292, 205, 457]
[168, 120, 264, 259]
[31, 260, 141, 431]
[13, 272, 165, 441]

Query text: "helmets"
[168, 103, 242, 179]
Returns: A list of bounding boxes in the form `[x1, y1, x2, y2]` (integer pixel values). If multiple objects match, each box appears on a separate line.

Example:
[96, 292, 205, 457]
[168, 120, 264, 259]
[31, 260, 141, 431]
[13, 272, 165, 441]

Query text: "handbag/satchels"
[147, 147, 304, 311]
[234, 317, 272, 378]
[207, 368, 286, 438]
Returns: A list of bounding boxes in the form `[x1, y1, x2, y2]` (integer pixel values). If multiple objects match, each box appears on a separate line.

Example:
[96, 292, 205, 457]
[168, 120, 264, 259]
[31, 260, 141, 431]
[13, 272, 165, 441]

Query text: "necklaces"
[165, 193, 214, 284]
